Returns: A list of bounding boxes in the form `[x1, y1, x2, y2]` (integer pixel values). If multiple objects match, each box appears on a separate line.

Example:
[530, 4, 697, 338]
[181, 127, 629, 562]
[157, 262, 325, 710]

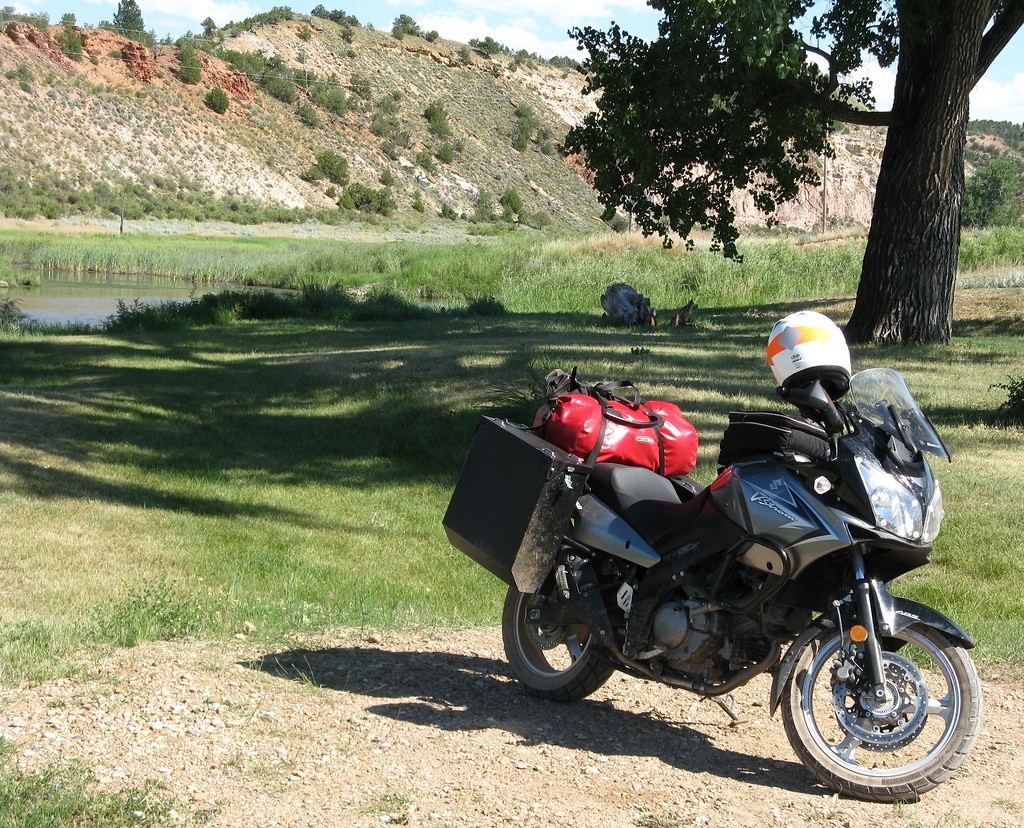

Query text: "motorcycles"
[501, 367, 984, 805]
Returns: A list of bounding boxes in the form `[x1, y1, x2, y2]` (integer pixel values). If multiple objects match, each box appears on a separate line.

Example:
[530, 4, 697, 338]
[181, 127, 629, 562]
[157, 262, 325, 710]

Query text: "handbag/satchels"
[718, 410, 831, 466]
[530, 397, 698, 478]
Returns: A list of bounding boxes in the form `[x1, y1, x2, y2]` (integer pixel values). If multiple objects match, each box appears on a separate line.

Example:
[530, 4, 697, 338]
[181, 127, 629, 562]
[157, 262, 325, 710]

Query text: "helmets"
[767, 311, 851, 407]
